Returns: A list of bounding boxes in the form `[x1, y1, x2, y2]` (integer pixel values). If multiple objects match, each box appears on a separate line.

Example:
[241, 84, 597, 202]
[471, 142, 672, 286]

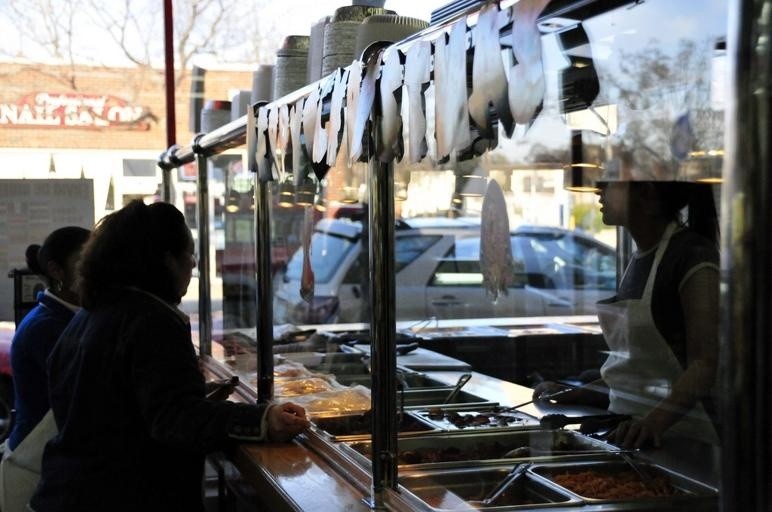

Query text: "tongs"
[540, 413, 631, 444]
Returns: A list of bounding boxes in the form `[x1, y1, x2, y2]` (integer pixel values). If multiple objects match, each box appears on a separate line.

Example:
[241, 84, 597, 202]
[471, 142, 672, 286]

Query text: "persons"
[28, 196, 310, 509]
[530, 117, 720, 496]
[1, 227, 241, 512]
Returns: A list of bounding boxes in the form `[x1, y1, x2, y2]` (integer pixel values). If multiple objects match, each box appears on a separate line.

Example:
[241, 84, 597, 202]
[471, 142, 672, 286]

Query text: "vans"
[272, 210, 732, 322]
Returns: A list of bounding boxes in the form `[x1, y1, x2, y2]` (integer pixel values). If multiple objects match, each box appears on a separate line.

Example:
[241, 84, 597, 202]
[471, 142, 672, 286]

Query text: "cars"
[0, 320, 20, 445]
[185, 192, 330, 298]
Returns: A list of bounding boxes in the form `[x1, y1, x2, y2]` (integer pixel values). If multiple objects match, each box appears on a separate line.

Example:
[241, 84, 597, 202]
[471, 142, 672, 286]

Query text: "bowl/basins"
[199, 3, 429, 134]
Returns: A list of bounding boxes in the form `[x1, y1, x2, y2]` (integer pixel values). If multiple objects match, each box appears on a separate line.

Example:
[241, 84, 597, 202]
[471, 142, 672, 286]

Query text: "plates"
[329, 330, 419, 356]
